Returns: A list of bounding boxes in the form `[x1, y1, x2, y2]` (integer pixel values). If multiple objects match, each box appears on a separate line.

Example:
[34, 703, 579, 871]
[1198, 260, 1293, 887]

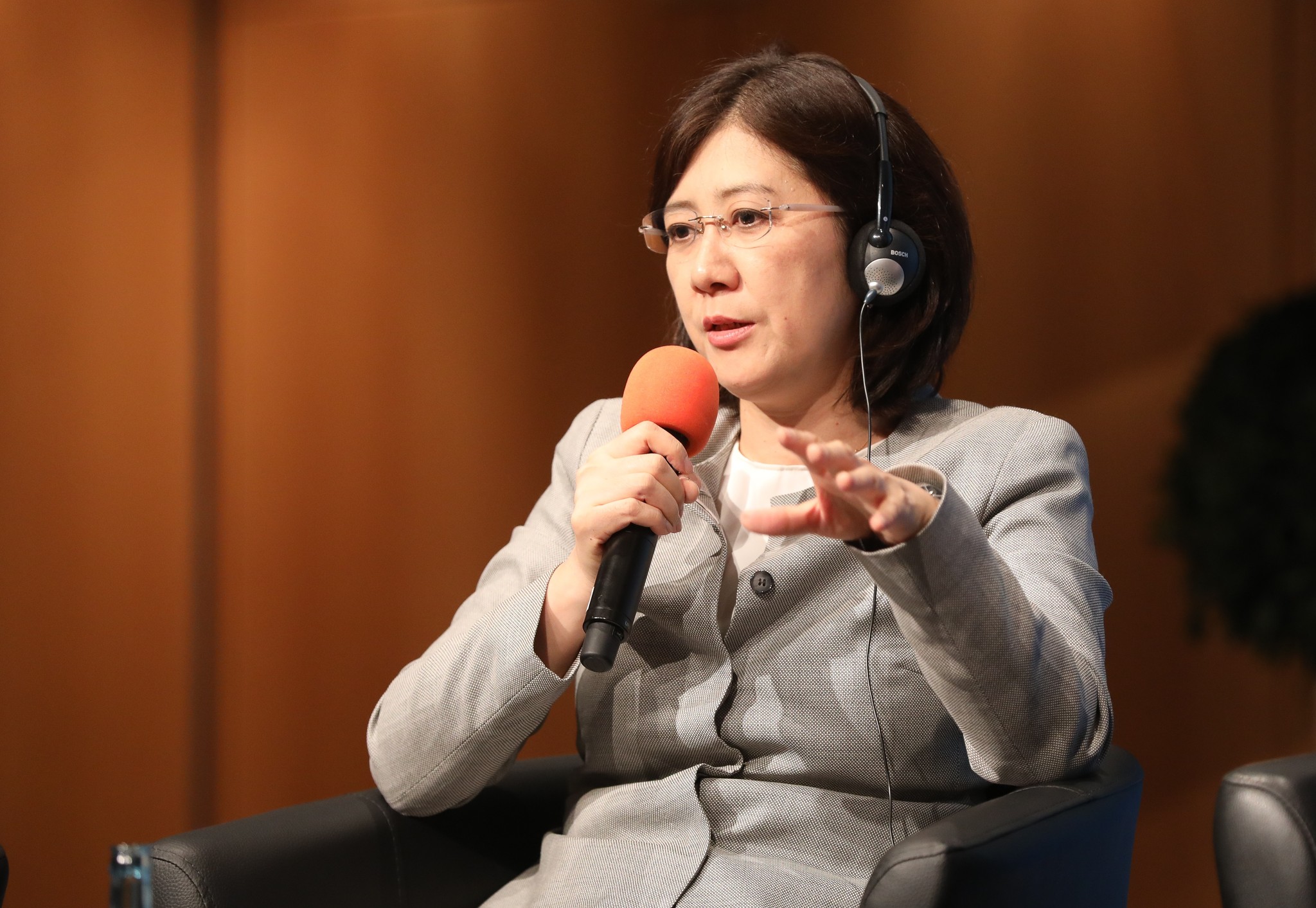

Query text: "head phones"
[849, 76, 927, 310]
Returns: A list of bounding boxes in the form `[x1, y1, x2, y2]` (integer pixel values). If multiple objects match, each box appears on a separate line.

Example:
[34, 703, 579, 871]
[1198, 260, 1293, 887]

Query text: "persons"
[364, 52, 1113, 908]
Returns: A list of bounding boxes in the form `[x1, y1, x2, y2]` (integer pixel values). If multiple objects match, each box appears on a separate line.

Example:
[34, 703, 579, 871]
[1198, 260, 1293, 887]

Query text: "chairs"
[109, 740, 1141, 908]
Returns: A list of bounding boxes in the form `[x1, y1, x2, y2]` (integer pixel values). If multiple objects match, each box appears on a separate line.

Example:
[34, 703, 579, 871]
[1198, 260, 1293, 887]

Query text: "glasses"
[638, 199, 846, 255]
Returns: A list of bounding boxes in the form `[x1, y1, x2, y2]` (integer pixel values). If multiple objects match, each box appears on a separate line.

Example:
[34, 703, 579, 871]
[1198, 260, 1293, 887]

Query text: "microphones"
[578, 344, 719, 670]
